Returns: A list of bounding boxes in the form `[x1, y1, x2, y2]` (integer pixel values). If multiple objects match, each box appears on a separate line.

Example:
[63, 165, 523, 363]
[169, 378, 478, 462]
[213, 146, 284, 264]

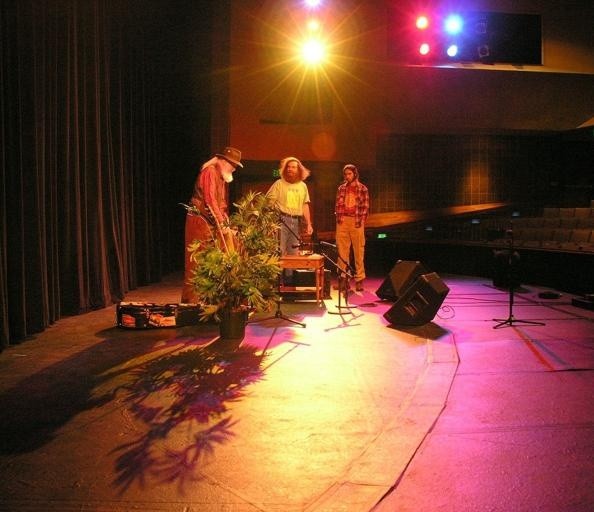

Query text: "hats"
[216, 147, 243, 169]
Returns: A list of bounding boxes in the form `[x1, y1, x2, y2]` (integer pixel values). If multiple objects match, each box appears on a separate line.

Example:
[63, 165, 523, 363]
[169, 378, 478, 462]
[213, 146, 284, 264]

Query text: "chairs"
[480, 200, 594, 252]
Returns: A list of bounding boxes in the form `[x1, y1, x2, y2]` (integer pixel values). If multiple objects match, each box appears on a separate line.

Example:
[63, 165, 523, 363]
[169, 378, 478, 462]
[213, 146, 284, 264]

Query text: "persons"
[266, 156, 314, 304]
[181, 147, 244, 304]
[332, 164, 370, 291]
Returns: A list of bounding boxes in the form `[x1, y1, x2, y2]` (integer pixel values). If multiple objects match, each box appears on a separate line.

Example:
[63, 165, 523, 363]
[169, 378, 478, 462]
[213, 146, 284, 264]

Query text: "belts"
[281, 211, 302, 219]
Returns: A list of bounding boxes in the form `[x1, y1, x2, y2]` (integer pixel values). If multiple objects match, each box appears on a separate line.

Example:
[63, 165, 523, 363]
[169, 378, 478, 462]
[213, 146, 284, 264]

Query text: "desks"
[278, 254, 325, 303]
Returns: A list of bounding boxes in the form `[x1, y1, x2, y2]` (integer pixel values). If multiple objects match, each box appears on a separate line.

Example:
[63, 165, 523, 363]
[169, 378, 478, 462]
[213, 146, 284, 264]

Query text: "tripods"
[492, 222, 545, 329]
[245, 208, 308, 327]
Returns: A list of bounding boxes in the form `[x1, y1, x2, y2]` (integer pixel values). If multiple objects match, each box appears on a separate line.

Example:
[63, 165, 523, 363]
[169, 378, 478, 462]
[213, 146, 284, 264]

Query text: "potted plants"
[187, 224, 286, 340]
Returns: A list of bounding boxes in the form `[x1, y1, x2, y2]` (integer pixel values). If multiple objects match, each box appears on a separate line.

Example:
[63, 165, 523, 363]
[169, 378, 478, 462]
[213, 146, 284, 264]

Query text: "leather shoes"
[356, 281, 363, 290]
[334, 279, 347, 290]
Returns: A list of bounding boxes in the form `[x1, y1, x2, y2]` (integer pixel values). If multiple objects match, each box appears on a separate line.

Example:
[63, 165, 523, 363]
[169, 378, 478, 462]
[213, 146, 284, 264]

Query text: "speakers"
[376, 260, 429, 302]
[383, 272, 449, 326]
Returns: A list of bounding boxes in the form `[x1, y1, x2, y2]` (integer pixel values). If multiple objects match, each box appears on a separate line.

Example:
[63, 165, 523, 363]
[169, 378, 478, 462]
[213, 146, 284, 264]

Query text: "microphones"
[291, 243, 312, 248]
[320, 241, 337, 248]
